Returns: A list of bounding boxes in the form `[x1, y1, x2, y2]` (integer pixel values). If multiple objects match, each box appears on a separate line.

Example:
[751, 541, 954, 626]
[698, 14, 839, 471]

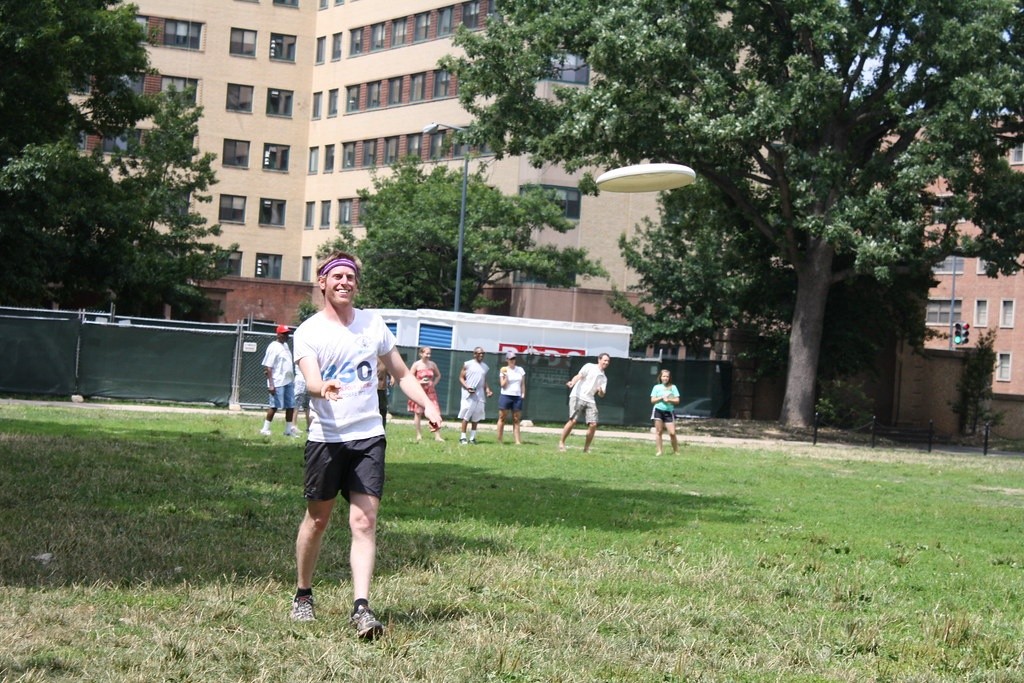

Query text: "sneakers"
[291, 592, 315, 621]
[349, 604, 383, 641]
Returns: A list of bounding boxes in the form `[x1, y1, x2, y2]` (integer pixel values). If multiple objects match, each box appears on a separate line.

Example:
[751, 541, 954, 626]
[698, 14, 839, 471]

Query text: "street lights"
[422, 121, 469, 312]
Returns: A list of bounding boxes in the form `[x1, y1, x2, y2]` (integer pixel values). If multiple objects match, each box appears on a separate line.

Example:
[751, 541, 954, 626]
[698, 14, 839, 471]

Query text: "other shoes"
[284, 432, 300, 438]
[291, 427, 303, 433]
[260, 430, 271, 435]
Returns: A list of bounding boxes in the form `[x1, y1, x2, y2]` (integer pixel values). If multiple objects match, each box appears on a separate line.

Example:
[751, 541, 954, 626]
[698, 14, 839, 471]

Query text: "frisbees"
[597, 163, 697, 193]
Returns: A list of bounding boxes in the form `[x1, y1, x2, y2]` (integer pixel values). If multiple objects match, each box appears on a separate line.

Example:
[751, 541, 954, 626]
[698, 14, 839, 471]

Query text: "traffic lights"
[960, 323, 970, 344]
[955, 323, 962, 344]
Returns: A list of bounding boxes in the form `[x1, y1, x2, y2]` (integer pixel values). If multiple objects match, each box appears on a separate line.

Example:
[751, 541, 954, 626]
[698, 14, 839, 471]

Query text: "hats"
[277, 325, 291, 333]
[506, 353, 516, 359]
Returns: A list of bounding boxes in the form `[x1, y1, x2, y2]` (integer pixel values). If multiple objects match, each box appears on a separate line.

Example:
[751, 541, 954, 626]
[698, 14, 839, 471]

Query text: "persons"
[496, 351, 527, 445]
[408, 346, 446, 440]
[458, 347, 494, 445]
[559, 352, 611, 454]
[261, 322, 310, 438]
[291, 249, 443, 640]
[376, 353, 395, 428]
[649, 369, 680, 456]
